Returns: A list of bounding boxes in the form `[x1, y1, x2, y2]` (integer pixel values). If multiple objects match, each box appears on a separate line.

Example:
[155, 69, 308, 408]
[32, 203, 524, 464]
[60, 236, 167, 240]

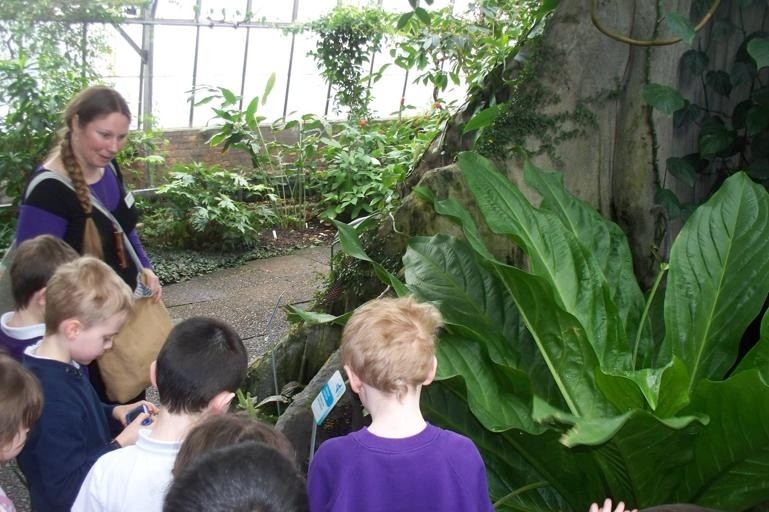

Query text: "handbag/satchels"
[96, 269, 175, 404]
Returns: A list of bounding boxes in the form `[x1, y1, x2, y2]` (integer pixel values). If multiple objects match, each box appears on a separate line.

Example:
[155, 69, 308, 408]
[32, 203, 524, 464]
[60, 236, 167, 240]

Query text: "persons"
[0, 232, 80, 363]
[65, 314, 246, 511]
[303, 292, 499, 512]
[15, 254, 166, 511]
[11, 82, 165, 435]
[171, 412, 294, 477]
[584, 497, 720, 511]
[154, 438, 310, 511]
[1, 345, 48, 511]
